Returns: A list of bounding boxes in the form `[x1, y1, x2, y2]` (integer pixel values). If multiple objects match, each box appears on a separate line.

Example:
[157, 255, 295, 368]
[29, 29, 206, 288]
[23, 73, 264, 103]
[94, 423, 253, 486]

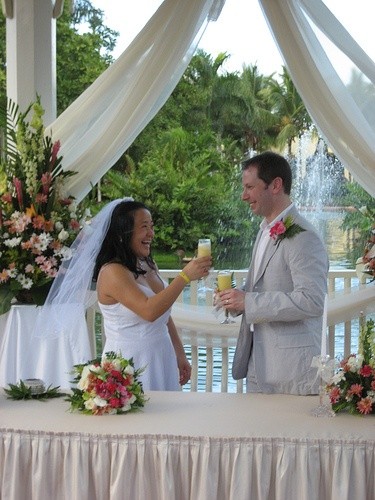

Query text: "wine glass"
[218, 269, 236, 324]
[198, 239, 214, 291]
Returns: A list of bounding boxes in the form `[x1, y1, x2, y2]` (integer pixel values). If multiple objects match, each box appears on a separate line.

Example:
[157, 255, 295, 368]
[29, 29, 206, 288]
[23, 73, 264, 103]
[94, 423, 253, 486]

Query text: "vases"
[14, 277, 55, 306]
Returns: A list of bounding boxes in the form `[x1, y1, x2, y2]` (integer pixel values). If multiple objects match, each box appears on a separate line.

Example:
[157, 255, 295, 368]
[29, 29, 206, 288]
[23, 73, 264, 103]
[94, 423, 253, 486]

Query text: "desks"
[0, 288, 99, 395]
[0, 391, 375, 500]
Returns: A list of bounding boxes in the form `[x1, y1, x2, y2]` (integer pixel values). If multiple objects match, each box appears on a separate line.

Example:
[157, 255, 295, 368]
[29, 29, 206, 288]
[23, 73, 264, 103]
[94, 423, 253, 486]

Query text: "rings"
[226, 299, 229, 304]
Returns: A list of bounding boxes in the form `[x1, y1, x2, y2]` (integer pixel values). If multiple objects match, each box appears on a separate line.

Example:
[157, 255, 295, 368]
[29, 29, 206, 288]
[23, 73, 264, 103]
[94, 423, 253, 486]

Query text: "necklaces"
[136, 261, 148, 277]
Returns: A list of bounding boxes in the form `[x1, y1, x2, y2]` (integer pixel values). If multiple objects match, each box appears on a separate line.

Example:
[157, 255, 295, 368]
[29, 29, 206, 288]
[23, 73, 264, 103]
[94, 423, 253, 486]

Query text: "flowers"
[0, 91, 86, 311]
[65, 350, 153, 417]
[268, 216, 306, 248]
[309, 308, 375, 418]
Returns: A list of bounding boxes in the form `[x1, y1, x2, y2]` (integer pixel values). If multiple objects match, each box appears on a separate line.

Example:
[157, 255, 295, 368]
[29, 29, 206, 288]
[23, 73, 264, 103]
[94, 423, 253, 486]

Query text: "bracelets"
[178, 272, 190, 283]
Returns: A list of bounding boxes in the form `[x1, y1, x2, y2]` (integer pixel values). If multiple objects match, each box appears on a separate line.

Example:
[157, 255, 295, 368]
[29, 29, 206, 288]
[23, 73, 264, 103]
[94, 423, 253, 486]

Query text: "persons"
[213, 151, 329, 397]
[87, 197, 212, 391]
